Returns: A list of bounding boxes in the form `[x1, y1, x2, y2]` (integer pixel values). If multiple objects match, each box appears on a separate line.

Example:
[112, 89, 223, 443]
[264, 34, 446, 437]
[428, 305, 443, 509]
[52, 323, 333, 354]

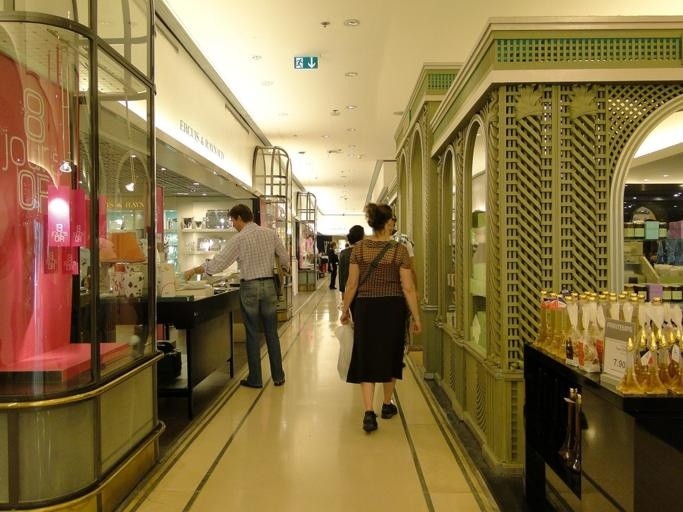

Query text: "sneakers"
[381, 403, 397, 419]
[363, 411, 377, 431]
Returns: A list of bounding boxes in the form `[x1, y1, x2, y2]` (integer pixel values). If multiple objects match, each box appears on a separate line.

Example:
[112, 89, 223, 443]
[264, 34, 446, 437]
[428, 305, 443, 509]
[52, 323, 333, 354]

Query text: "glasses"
[392, 217, 396, 223]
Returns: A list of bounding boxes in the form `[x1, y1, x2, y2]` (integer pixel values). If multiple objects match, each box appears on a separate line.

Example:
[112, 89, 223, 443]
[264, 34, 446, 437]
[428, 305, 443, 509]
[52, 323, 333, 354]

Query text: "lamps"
[99, 233, 146, 297]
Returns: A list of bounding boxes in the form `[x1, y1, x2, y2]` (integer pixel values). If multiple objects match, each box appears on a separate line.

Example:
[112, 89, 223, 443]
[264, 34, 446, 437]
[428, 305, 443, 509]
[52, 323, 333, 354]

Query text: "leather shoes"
[274, 379, 285, 386]
[240, 379, 263, 388]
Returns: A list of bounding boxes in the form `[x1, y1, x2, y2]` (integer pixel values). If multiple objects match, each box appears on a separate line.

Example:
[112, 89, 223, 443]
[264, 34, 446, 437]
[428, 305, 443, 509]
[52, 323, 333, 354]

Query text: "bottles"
[622, 220, 666, 239]
[538, 290, 682, 397]
[624, 282, 682, 302]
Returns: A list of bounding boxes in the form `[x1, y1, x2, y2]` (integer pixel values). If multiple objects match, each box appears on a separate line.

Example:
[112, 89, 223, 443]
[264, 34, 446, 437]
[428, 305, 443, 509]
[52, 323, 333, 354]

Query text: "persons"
[328, 202, 422, 430]
[183, 203, 289, 388]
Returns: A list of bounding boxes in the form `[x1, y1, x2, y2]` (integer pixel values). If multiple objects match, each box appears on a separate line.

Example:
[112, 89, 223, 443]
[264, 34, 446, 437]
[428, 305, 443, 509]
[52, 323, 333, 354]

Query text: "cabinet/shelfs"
[523, 340, 683, 512]
[80, 288, 241, 420]
[181, 229, 238, 256]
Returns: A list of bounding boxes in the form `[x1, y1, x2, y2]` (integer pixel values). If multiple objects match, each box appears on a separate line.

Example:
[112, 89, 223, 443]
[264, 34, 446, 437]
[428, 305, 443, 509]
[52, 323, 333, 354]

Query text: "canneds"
[624, 221, 668, 239]
[624, 283, 682, 302]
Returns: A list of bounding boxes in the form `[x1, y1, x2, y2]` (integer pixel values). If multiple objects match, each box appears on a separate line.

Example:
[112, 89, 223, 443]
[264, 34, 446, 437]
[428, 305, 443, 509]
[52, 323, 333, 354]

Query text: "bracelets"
[192, 268, 196, 274]
